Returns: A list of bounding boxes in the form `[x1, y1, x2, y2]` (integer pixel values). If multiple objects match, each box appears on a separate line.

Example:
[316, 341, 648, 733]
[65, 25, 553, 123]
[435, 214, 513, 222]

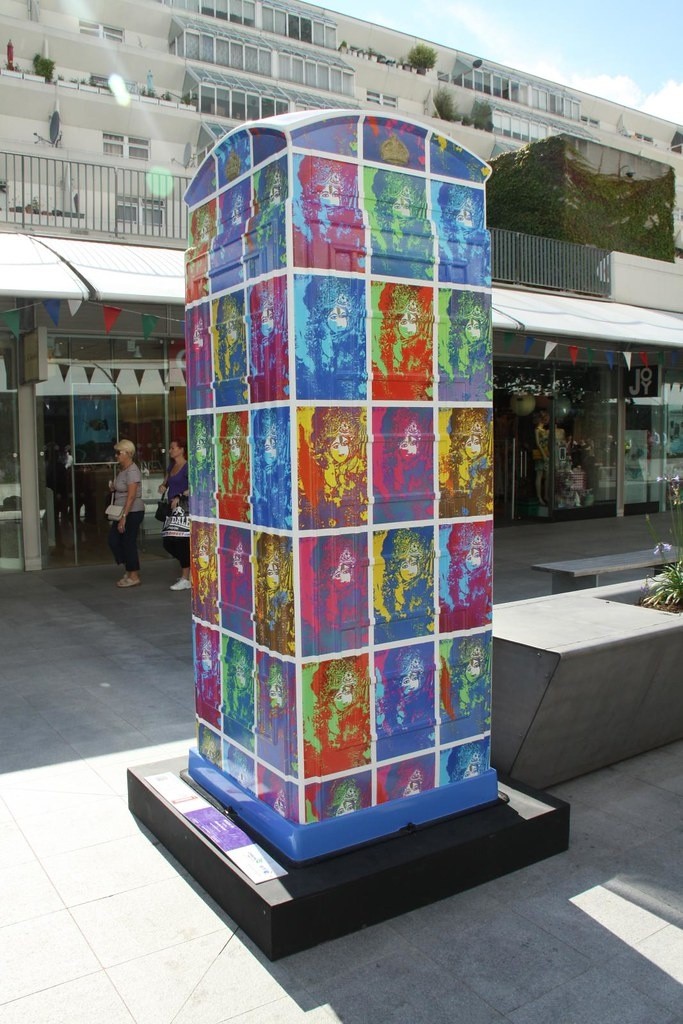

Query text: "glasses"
[114, 450, 127, 456]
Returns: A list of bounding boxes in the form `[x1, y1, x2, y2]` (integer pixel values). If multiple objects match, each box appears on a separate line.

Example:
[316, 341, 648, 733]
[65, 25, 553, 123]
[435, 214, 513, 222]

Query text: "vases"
[352, 51, 358, 56]
[25, 206, 31, 213]
[42, 212, 52, 216]
[16, 206, 22, 212]
[369, 55, 377, 62]
[9, 208, 14, 212]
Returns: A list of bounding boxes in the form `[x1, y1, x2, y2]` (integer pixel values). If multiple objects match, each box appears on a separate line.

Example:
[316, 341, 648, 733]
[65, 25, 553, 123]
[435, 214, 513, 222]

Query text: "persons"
[157, 439, 191, 590]
[533, 410, 551, 507]
[106, 439, 145, 587]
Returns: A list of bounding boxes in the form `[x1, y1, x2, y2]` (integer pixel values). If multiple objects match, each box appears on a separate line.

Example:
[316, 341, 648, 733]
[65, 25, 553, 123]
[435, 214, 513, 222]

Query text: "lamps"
[132, 345, 143, 359]
[53, 343, 63, 357]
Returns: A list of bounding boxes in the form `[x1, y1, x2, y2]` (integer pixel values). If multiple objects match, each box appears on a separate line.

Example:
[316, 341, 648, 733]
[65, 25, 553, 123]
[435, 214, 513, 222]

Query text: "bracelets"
[120, 513, 126, 518]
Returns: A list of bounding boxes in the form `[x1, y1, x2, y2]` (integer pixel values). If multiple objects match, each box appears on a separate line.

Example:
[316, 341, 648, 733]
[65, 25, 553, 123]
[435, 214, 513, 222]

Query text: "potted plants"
[397, 57, 404, 69]
[386, 60, 392, 65]
[406, 41, 440, 75]
[33, 197, 39, 214]
[338, 40, 348, 53]
[347, 46, 359, 55]
[363, 52, 369, 60]
[393, 61, 396, 67]
[411, 65, 417, 74]
[358, 50, 364, 58]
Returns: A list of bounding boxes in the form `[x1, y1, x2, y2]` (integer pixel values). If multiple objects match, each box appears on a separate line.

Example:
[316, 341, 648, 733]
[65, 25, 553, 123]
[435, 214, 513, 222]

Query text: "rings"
[120, 526, 123, 528]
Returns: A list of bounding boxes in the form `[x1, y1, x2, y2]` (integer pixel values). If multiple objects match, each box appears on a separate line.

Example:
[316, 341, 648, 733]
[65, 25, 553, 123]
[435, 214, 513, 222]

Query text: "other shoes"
[170, 576, 191, 590]
[116, 574, 141, 588]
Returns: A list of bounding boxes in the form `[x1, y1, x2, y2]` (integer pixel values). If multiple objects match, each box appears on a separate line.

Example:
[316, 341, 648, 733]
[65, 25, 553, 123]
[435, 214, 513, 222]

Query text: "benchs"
[531, 545, 683, 594]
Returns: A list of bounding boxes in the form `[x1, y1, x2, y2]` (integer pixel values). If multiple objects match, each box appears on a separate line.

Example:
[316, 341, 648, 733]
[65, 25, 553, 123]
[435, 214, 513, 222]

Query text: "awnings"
[0, 232, 683, 351]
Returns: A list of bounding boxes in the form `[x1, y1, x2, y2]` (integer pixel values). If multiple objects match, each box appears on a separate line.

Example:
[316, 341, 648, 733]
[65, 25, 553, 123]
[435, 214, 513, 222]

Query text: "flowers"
[368, 48, 377, 57]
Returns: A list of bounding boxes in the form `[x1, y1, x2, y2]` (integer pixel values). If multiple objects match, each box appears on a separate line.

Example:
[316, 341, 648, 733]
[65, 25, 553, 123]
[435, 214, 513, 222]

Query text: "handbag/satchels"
[155, 502, 173, 523]
[104, 505, 124, 521]
[533, 448, 550, 459]
